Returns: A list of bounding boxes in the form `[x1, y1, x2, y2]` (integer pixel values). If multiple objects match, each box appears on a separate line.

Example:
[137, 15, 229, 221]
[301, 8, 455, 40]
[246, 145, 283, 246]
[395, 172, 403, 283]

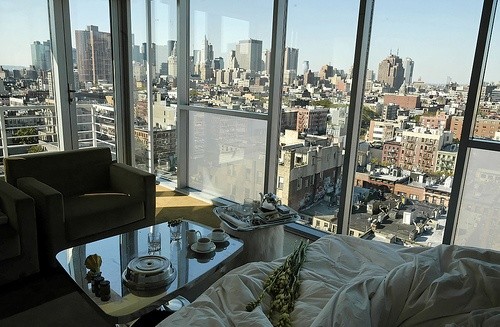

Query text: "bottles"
[91, 273, 111, 301]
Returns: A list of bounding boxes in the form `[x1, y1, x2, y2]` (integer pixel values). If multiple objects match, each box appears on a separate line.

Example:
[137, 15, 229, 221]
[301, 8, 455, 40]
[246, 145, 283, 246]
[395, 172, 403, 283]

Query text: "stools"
[218, 222, 284, 270]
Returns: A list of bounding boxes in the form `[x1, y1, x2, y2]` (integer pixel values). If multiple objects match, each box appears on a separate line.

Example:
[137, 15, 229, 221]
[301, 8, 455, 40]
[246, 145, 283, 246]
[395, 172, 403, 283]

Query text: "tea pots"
[259, 191, 282, 212]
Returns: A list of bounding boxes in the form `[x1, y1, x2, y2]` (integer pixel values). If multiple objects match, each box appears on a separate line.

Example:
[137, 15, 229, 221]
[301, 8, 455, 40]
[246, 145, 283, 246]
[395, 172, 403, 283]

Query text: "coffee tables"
[55, 218, 244, 327]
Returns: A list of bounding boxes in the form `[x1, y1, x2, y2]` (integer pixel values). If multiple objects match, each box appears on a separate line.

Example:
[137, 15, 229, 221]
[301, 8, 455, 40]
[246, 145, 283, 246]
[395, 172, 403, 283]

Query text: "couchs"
[0, 179, 40, 285]
[3, 146, 156, 270]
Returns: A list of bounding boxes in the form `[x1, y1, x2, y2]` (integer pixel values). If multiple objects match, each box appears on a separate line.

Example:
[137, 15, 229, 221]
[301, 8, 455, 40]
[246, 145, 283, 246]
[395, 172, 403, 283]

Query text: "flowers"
[246, 239, 310, 327]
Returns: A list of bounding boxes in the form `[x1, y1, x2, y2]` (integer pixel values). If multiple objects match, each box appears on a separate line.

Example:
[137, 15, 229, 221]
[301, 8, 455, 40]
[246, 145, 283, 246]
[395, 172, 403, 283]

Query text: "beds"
[154, 233, 500, 327]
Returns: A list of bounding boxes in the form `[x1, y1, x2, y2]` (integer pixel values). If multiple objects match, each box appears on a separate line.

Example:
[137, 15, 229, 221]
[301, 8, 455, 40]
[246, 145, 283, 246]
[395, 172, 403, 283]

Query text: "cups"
[197, 238, 214, 251]
[187, 229, 202, 244]
[212, 228, 226, 241]
[244, 197, 253, 212]
[147, 232, 162, 251]
[170, 224, 183, 240]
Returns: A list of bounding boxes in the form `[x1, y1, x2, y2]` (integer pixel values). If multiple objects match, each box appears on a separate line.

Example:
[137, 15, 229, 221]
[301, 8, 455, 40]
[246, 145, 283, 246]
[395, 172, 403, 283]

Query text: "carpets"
[0, 229, 315, 327]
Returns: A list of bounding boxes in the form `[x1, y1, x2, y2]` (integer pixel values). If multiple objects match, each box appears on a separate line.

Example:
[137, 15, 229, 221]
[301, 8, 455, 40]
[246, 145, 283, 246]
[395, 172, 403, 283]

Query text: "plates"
[190, 242, 217, 253]
[121, 255, 177, 289]
[206, 232, 230, 242]
